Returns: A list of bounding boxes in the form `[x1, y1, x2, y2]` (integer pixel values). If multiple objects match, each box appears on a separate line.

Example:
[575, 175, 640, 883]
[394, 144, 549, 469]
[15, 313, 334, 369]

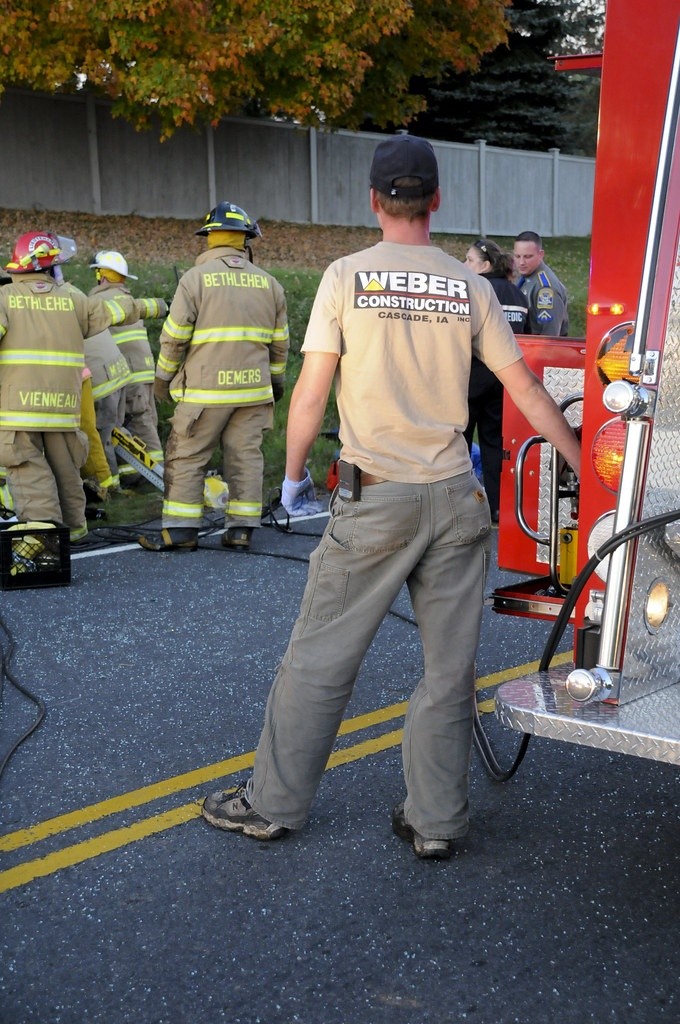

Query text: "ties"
[516, 275, 525, 289]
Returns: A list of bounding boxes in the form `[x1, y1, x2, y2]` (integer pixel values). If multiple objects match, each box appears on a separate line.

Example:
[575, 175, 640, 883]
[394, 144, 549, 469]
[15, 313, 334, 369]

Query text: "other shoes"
[221, 526, 252, 552]
[489, 510, 500, 522]
[84, 508, 105, 523]
[137, 528, 200, 553]
[82, 482, 106, 504]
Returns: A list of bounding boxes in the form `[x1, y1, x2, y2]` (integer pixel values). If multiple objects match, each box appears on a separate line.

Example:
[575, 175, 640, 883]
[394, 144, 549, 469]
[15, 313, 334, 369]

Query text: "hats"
[368, 133, 440, 197]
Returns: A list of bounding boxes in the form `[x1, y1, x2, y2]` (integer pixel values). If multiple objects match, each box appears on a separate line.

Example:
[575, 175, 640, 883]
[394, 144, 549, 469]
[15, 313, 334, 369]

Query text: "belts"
[358, 471, 387, 487]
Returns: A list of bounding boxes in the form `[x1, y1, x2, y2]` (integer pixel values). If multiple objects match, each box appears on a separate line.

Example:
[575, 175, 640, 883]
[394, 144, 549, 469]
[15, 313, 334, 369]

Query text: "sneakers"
[201, 780, 289, 843]
[393, 801, 453, 859]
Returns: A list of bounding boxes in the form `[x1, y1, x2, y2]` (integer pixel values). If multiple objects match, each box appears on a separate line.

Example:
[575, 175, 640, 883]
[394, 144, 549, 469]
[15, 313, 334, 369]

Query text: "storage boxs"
[0, 520, 71, 591]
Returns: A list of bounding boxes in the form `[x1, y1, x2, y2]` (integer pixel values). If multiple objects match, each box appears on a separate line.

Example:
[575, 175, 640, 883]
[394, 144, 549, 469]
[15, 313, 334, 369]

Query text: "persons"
[514, 231, 569, 337]
[137, 201, 289, 551]
[463, 240, 532, 526]
[0, 232, 170, 546]
[200, 137, 578, 858]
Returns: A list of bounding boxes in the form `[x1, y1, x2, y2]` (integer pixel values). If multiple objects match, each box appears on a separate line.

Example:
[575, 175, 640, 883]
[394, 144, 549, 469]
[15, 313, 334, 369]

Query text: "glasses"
[474, 239, 497, 265]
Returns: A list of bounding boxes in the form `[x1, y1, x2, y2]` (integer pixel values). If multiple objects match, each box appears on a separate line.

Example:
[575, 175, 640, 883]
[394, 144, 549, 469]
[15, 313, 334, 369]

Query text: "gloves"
[269, 382, 286, 403]
[153, 379, 172, 404]
[280, 465, 324, 517]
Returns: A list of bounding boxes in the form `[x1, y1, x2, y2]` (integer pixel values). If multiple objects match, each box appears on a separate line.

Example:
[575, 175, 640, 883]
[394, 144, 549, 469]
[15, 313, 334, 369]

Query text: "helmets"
[193, 201, 264, 239]
[2, 230, 69, 275]
[87, 248, 140, 280]
